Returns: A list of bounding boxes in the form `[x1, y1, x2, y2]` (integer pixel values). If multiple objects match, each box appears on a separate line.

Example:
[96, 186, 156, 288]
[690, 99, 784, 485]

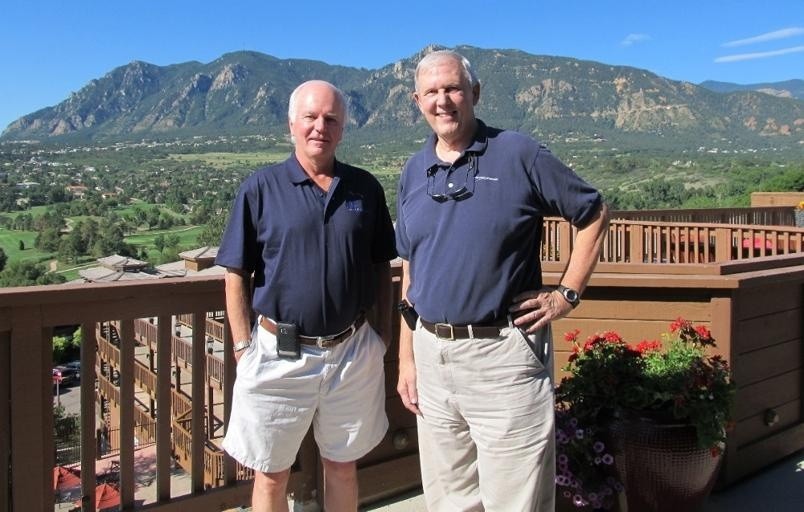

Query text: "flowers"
[551, 317, 733, 512]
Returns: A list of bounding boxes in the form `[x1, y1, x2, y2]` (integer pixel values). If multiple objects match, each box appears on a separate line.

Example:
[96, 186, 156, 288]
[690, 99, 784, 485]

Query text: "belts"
[419, 311, 523, 341]
[260, 315, 366, 348]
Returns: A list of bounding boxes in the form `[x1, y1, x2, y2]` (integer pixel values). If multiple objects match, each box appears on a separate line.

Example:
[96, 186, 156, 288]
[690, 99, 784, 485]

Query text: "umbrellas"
[76, 482, 121, 512]
[53, 464, 82, 510]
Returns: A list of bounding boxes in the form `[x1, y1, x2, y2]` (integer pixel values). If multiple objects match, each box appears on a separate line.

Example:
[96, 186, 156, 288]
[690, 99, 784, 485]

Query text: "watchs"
[558, 284, 580, 311]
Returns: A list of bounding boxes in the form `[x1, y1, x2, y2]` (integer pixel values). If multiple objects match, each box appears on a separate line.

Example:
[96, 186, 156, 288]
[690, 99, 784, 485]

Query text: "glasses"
[427, 158, 471, 202]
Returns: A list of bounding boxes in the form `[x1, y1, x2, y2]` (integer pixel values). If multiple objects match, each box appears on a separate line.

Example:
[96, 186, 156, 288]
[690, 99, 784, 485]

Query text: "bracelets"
[231, 339, 252, 352]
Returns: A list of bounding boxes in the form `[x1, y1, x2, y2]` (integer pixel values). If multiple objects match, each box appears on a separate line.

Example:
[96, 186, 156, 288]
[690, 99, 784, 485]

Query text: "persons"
[387, 49, 608, 512]
[211, 77, 395, 512]
